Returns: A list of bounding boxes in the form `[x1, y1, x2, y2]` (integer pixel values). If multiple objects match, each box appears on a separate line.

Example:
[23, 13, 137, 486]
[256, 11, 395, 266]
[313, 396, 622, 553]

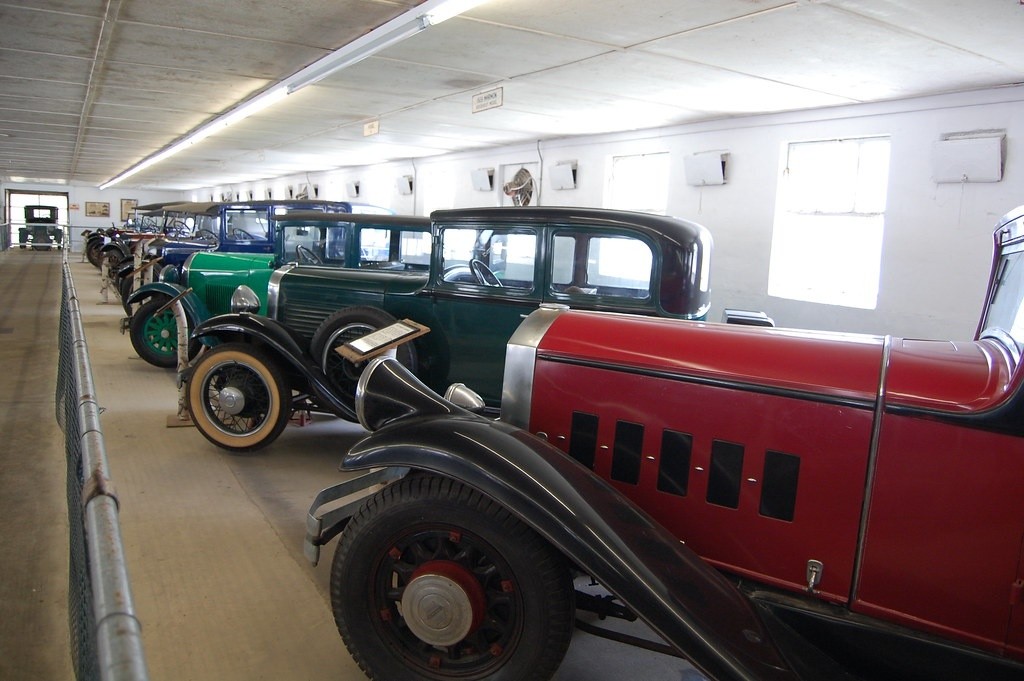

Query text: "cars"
[83, 200, 353, 368]
[307, 201, 1024, 681]
[19, 205, 62, 251]
[177, 167, 776, 455]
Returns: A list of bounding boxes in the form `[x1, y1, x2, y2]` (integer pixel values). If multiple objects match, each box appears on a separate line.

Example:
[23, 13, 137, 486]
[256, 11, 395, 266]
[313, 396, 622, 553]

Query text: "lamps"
[427, 0, 490, 26]
[287, 16, 426, 94]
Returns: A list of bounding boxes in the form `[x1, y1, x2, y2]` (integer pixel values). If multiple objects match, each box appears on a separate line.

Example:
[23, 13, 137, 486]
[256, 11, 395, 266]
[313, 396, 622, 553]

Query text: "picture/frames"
[121, 199, 138, 222]
[85, 202, 110, 217]
